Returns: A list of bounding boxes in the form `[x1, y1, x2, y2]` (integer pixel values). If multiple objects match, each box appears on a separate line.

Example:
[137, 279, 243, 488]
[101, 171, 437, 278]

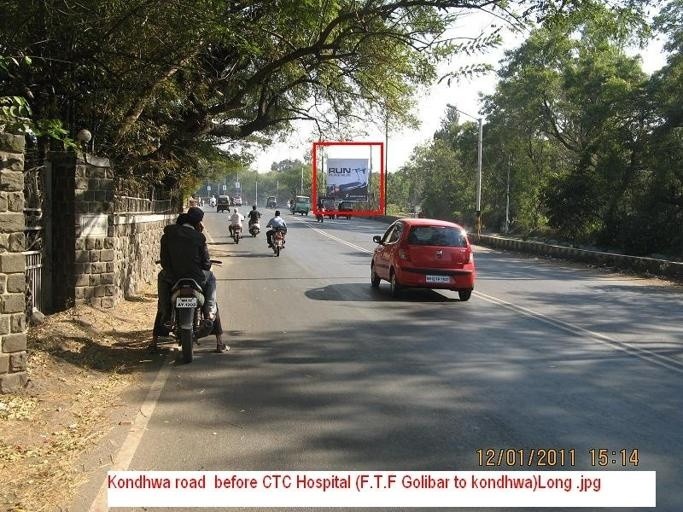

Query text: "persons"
[248, 205, 263, 228]
[229, 208, 245, 238]
[148, 207, 230, 354]
[266, 211, 288, 249]
[316, 200, 326, 210]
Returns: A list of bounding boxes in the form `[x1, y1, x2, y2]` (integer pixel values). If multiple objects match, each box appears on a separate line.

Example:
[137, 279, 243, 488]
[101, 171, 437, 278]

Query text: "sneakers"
[147, 344, 156, 353]
[204, 314, 216, 322]
[216, 343, 230, 352]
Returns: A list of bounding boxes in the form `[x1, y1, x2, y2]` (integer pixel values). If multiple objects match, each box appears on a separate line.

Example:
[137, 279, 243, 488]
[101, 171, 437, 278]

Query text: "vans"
[289, 194, 309, 215]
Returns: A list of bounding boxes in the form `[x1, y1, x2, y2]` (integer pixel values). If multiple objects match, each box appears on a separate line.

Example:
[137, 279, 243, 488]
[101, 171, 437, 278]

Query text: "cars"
[327, 183, 367, 201]
[217, 195, 230, 212]
[265, 196, 278, 209]
[369, 218, 477, 301]
[234, 193, 243, 205]
[338, 201, 354, 219]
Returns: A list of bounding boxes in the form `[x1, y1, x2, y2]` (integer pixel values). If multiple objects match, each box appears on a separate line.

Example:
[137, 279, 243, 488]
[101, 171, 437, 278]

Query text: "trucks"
[318, 196, 336, 219]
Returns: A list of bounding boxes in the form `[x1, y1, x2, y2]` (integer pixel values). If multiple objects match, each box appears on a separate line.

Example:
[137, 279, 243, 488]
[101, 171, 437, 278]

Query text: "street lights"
[446, 103, 482, 234]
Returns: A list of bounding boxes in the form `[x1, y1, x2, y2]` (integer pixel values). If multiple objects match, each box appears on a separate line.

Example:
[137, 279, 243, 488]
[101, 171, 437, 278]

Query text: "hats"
[187, 207, 205, 221]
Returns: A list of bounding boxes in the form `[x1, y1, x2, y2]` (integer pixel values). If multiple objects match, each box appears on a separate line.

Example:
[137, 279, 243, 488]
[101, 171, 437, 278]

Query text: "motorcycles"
[154, 255, 223, 362]
[316, 208, 324, 223]
[228, 215, 261, 245]
[268, 226, 284, 256]
